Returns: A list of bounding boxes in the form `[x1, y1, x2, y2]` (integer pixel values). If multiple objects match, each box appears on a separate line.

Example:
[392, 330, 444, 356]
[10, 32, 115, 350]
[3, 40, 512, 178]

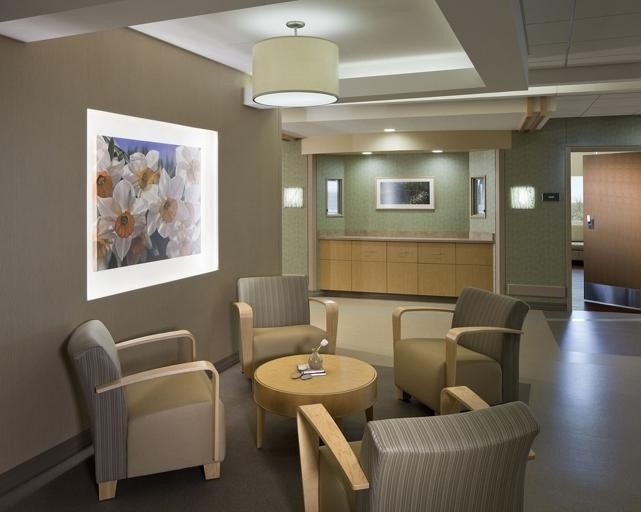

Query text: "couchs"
[233, 275, 339, 381]
[392, 286, 530, 416]
[572, 224, 584, 260]
[67, 318, 227, 502]
[298, 385, 541, 512]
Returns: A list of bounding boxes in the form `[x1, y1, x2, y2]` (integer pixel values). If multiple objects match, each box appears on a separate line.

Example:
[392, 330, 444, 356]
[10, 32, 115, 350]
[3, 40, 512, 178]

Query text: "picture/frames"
[375, 176, 436, 210]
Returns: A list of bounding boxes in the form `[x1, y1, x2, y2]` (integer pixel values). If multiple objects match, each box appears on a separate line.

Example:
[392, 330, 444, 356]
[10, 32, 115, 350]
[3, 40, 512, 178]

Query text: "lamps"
[251, 21, 340, 108]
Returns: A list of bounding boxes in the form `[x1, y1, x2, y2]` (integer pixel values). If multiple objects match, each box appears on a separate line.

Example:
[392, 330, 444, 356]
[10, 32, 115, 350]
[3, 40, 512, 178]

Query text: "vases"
[308, 350, 323, 369]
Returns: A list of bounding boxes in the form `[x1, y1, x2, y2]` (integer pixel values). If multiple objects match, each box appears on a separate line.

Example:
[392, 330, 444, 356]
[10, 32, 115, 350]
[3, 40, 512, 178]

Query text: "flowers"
[311, 338, 328, 352]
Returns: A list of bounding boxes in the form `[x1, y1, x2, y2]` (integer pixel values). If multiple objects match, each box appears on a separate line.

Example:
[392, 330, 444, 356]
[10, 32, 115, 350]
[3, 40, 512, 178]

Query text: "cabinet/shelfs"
[418, 242, 455, 298]
[319, 240, 352, 291]
[455, 242, 494, 298]
[351, 240, 386, 293]
[386, 242, 418, 295]
